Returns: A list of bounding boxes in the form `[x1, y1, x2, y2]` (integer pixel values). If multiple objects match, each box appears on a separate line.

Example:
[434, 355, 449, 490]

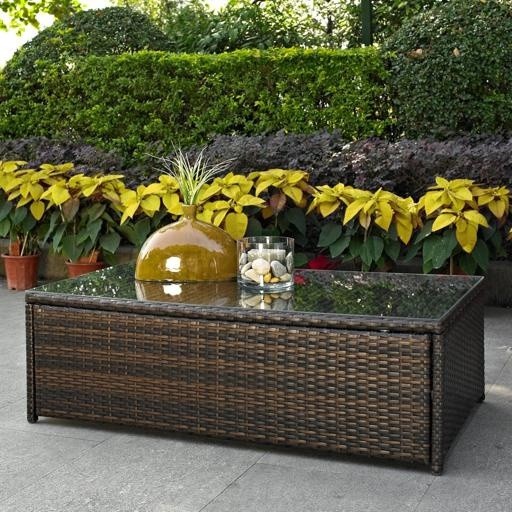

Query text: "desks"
[24, 257, 484, 476]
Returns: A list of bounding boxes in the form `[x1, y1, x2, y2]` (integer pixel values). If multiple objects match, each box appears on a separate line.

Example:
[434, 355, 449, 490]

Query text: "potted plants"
[135, 139, 240, 282]
[54, 163, 119, 280]
[0, 161, 53, 290]
